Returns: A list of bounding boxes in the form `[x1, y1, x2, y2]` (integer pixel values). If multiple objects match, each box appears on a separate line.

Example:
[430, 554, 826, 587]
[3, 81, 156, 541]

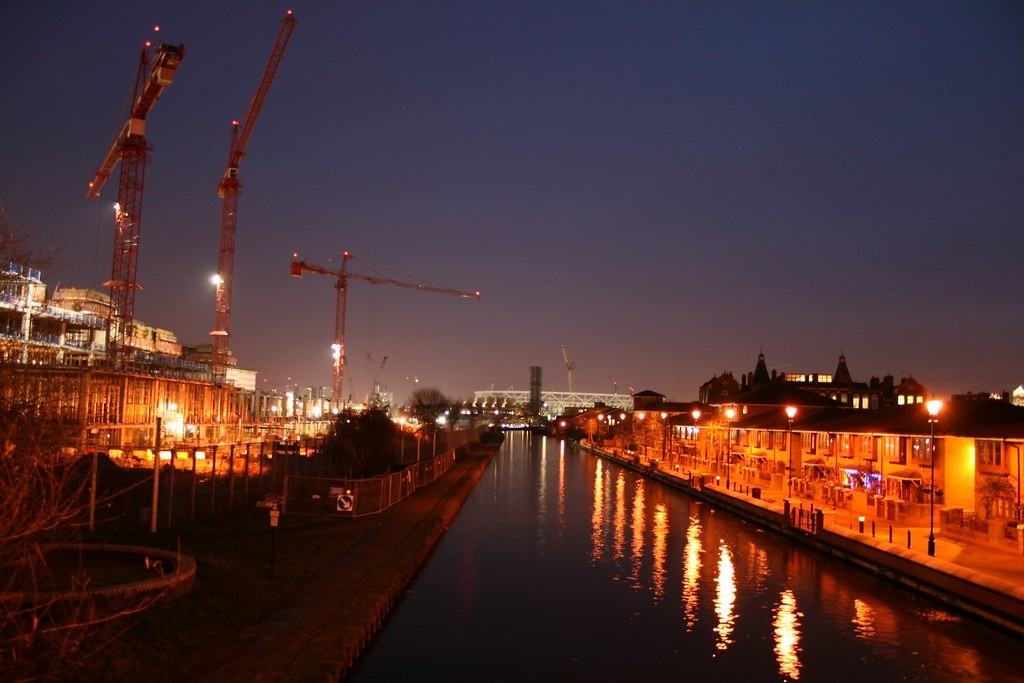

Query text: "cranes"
[208, 7, 300, 387]
[288, 251, 483, 404]
[83, 24, 185, 377]
[560, 343, 576, 394]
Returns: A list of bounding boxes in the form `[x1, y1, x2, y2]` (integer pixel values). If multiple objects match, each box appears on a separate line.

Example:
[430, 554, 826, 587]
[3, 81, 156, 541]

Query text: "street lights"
[598, 414, 605, 447]
[638, 413, 645, 454]
[660, 412, 668, 460]
[620, 413, 625, 453]
[784, 407, 797, 503]
[725, 408, 734, 488]
[691, 410, 701, 470]
[925, 400, 942, 558]
